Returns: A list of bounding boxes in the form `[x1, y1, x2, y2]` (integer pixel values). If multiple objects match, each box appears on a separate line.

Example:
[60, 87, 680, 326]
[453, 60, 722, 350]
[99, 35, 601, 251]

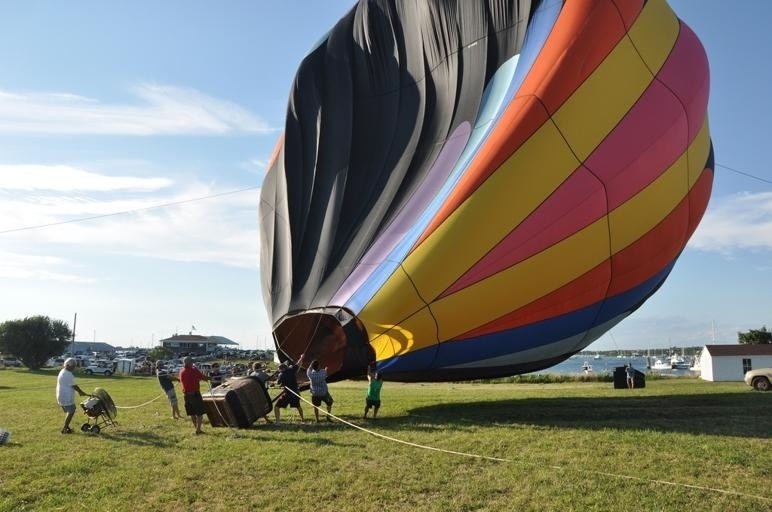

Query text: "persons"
[221, 360, 270, 376]
[55, 358, 91, 433]
[273, 354, 308, 427]
[156, 360, 183, 419]
[625, 363, 634, 389]
[179, 357, 208, 434]
[307, 360, 333, 422]
[209, 363, 226, 389]
[364, 364, 384, 419]
[249, 363, 273, 423]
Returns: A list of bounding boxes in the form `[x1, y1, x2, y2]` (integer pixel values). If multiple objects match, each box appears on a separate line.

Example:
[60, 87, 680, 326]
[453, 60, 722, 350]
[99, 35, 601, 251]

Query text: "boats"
[646, 353, 690, 370]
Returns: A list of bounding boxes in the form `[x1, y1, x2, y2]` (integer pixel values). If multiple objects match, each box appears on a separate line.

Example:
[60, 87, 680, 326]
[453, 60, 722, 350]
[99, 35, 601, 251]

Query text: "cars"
[161, 363, 248, 378]
[3, 357, 21, 367]
[44, 356, 155, 376]
[744, 368, 772, 391]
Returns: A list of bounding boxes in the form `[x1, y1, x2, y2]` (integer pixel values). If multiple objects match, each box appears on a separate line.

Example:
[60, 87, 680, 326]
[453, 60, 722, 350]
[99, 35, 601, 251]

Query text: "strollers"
[79, 387, 119, 434]
